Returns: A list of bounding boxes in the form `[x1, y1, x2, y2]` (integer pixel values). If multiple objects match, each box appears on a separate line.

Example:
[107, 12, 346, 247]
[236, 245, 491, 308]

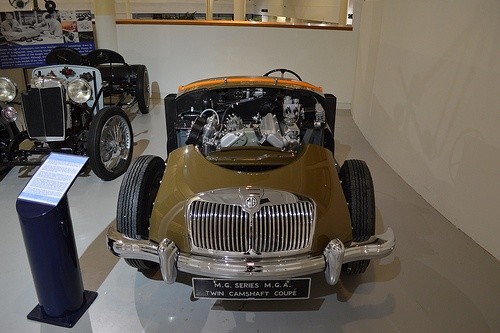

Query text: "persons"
[33, 12, 61, 35]
[1, 13, 21, 32]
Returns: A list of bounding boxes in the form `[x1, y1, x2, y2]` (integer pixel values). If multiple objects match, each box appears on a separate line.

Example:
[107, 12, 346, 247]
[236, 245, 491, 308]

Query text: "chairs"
[82, 48, 127, 85]
[43, 46, 84, 66]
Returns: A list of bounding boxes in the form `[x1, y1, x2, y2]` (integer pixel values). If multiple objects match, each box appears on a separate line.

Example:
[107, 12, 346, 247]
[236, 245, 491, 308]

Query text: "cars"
[0, 46, 151, 182]
[107, 68, 397, 301]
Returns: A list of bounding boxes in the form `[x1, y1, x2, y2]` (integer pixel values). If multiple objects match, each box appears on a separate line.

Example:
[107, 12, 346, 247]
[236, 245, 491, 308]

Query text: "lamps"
[9, 0, 57, 12]
